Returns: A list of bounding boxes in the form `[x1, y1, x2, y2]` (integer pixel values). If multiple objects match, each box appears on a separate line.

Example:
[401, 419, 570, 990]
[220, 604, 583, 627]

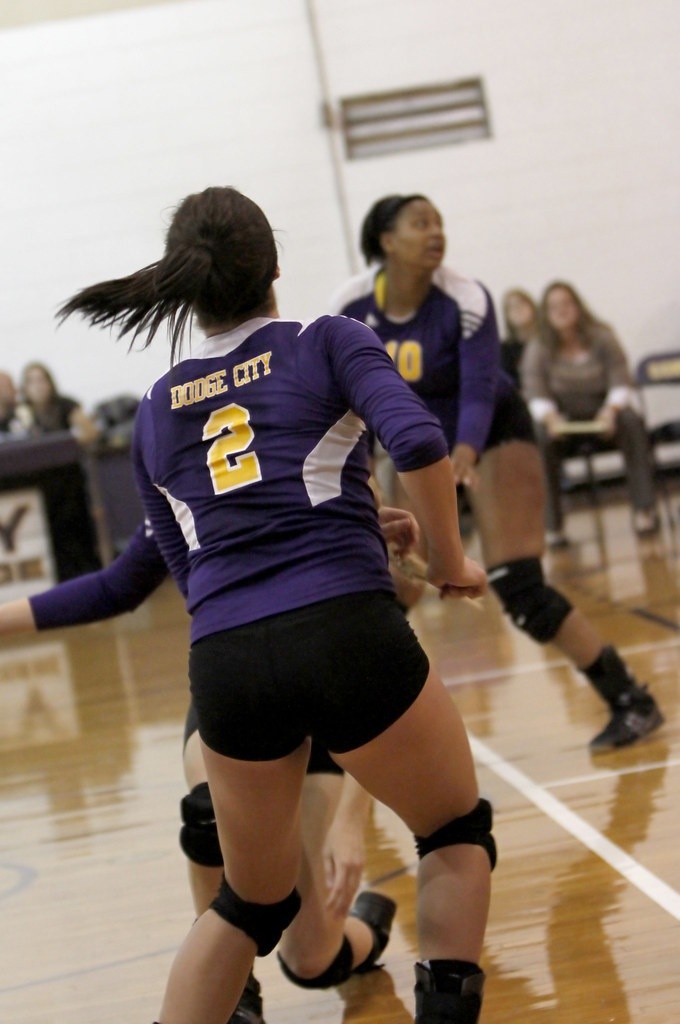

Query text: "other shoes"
[414, 959, 486, 1024]
[546, 531, 568, 551]
[355, 891, 397, 973]
[630, 504, 660, 538]
[230, 967, 266, 1024]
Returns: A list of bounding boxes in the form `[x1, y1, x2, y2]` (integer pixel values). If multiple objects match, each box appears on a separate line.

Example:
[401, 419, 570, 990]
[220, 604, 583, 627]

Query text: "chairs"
[558, 350, 680, 536]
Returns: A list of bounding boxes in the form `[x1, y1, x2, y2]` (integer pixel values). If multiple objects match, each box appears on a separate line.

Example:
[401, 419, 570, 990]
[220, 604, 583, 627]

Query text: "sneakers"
[590, 683, 663, 752]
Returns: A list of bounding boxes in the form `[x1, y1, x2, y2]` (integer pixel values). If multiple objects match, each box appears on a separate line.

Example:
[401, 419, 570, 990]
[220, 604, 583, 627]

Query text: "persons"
[0, 364, 84, 443]
[65, 185, 495, 1023]
[1, 517, 397, 1024]
[501, 284, 661, 546]
[330, 193, 662, 752]
